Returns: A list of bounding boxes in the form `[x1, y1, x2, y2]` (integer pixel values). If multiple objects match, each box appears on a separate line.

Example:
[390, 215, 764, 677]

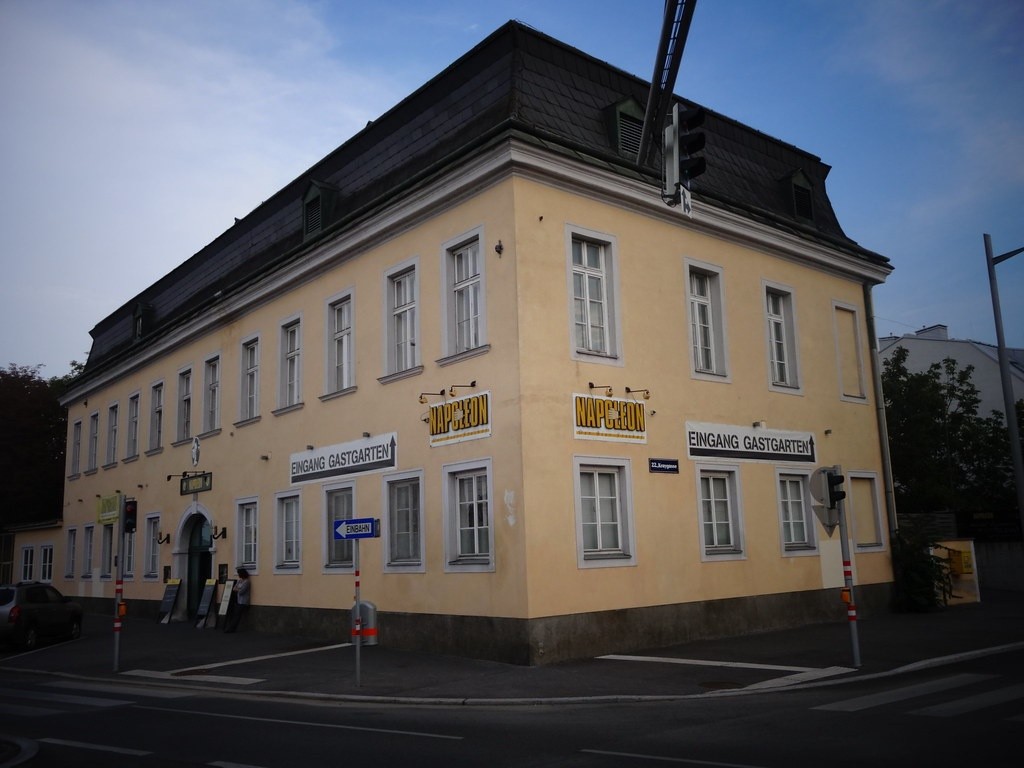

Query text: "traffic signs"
[333, 520, 375, 539]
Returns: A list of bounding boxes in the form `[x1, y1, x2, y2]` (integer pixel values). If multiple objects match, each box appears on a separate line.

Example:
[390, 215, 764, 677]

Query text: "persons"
[223, 568, 251, 633]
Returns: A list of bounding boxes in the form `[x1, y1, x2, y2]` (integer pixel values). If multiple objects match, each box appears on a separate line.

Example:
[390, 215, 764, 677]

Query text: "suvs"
[0, 581, 82, 647]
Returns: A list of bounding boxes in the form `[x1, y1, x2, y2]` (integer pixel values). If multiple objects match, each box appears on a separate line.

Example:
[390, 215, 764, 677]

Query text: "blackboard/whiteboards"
[196, 585, 216, 616]
[158, 584, 179, 612]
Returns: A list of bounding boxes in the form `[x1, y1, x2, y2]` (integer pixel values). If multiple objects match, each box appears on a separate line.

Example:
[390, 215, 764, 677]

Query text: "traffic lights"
[662, 101, 706, 206]
[126, 500, 137, 532]
